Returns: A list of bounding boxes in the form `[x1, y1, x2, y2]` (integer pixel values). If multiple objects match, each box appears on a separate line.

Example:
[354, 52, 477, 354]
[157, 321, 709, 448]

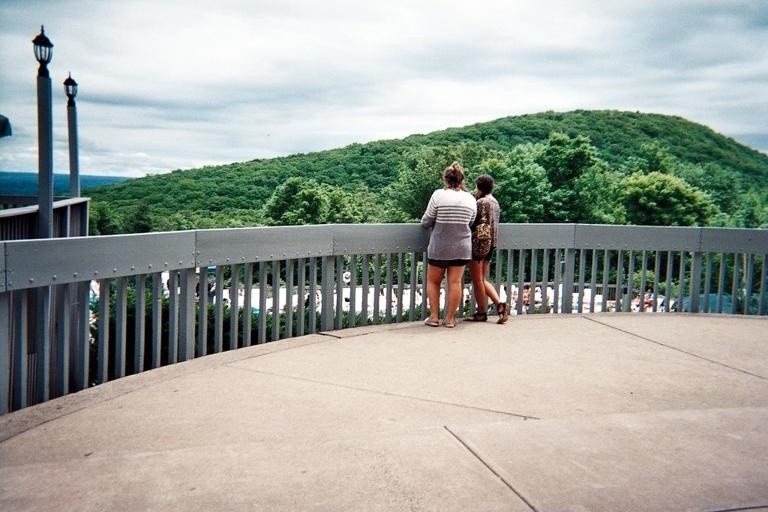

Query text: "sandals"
[497, 302, 510, 323]
[464, 310, 488, 321]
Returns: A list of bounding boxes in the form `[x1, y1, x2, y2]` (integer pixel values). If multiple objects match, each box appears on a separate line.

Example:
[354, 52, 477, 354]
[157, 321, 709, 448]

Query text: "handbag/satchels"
[473, 223, 492, 256]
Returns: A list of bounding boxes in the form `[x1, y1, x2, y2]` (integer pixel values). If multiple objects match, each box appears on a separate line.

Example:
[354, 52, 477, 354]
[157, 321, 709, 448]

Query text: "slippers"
[442, 317, 458, 326]
[424, 317, 439, 326]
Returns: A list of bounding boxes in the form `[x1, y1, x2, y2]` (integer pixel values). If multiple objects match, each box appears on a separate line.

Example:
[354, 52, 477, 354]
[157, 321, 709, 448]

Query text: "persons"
[644, 287, 655, 309]
[535, 289, 541, 304]
[465, 294, 471, 303]
[636, 295, 640, 307]
[513, 291, 518, 310]
[420, 161, 478, 329]
[463, 173, 510, 324]
